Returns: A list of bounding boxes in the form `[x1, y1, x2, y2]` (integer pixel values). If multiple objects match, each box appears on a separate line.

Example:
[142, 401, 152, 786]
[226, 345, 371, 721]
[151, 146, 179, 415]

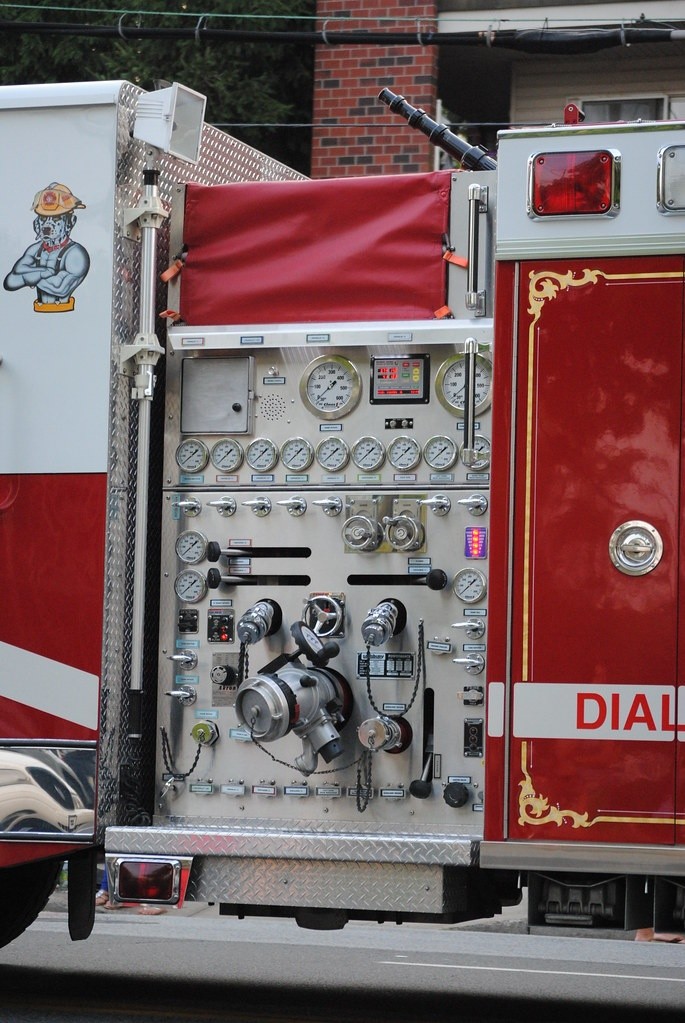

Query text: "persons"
[95, 863, 167, 915]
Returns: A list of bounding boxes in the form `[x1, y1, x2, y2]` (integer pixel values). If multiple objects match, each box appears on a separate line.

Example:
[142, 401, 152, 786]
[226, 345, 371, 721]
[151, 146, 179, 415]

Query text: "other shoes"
[95, 889, 109, 906]
[103, 901, 120, 910]
[139, 906, 167, 915]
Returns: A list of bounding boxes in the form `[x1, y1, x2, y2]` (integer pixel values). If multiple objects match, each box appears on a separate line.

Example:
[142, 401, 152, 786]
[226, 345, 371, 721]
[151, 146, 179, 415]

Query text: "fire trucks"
[0, 79, 685, 948]
